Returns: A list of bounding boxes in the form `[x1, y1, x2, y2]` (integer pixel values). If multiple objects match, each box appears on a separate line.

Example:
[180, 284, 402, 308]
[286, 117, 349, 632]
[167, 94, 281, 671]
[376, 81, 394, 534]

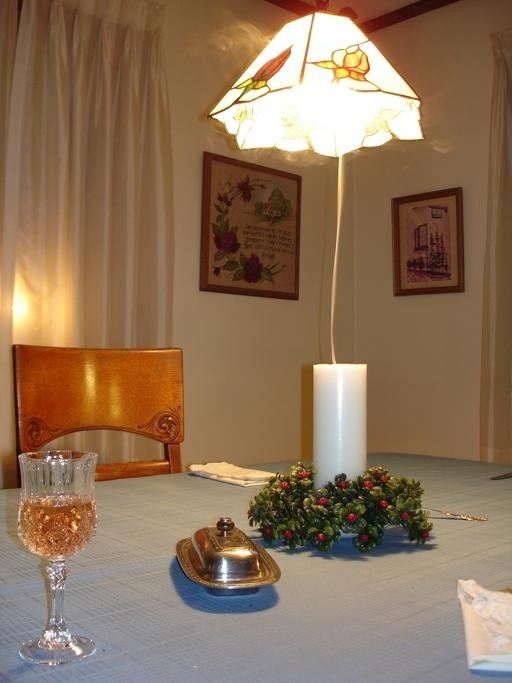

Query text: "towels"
[188, 461, 278, 489]
[457, 577, 512, 673]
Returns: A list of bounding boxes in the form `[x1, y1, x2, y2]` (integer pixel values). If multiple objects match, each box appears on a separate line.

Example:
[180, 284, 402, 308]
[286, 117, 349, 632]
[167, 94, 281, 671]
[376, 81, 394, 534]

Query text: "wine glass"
[16, 450, 99, 666]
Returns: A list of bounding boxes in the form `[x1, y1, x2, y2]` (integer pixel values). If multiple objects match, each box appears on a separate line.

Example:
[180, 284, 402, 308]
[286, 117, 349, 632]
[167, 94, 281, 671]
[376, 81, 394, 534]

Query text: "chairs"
[13, 344, 182, 488]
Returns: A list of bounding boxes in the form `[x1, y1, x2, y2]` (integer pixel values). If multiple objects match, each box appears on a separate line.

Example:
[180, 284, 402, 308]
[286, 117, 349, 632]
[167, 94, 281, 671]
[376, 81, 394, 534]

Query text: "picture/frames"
[393, 184, 466, 298]
[199, 151, 302, 302]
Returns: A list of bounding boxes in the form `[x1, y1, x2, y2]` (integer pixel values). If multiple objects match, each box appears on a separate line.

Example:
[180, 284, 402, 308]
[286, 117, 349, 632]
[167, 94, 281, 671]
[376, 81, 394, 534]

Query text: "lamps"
[206, 0, 424, 493]
